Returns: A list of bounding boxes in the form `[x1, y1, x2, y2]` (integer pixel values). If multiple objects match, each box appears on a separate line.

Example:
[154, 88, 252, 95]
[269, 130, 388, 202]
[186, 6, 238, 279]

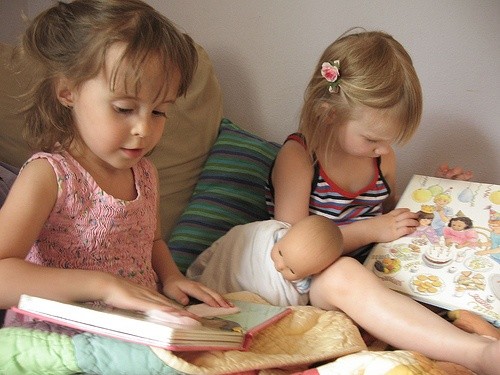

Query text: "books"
[363, 174, 499, 325]
[11, 291, 292, 352]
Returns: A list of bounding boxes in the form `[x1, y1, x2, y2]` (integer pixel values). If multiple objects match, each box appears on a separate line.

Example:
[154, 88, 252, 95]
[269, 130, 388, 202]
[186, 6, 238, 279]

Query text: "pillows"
[165, 117, 285, 275]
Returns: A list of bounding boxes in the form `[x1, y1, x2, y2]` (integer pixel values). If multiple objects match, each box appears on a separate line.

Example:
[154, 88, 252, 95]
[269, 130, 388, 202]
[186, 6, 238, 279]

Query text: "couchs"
[0, 30, 499, 375]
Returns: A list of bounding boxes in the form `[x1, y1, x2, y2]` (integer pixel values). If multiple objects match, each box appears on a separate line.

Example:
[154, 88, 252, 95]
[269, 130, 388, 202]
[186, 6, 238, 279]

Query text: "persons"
[0, 0, 226, 321]
[266, 27, 500, 375]
[200, 216, 344, 306]
